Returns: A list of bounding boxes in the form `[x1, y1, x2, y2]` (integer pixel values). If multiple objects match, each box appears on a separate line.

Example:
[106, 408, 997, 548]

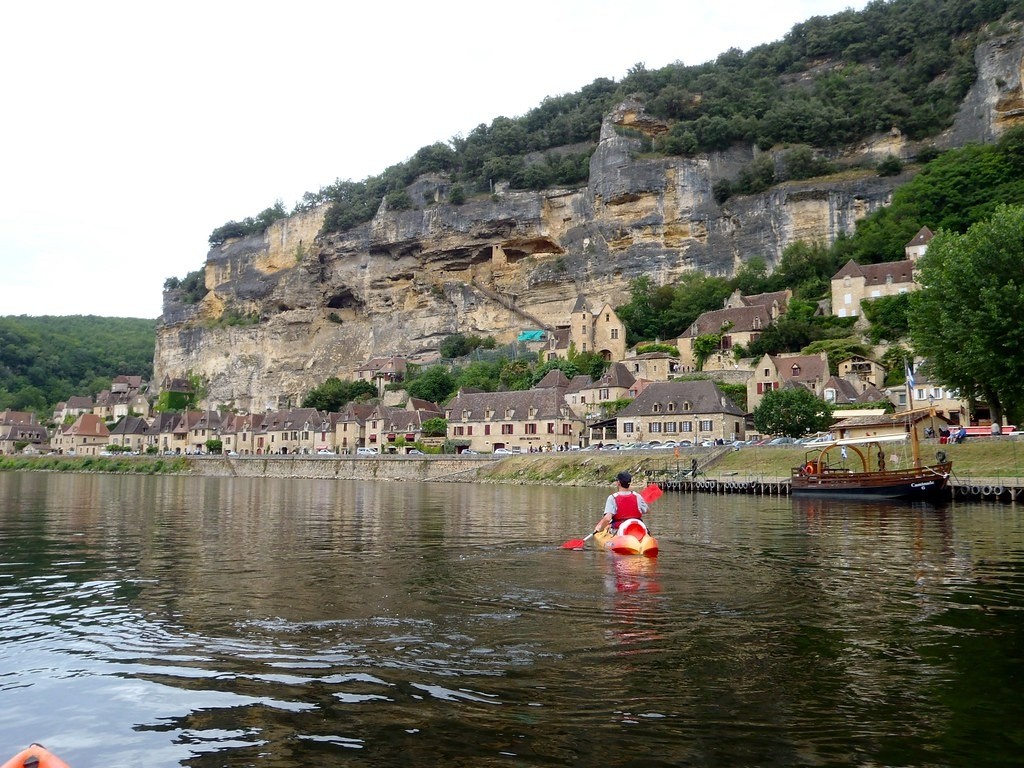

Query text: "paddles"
[557, 483, 663, 549]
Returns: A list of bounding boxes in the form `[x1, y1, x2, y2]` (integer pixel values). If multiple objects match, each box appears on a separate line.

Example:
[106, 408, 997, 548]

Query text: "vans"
[165, 451, 178, 455]
[357, 447, 378, 455]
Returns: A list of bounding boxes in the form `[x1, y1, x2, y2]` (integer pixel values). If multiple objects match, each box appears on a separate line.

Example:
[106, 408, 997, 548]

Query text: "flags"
[907, 367, 915, 388]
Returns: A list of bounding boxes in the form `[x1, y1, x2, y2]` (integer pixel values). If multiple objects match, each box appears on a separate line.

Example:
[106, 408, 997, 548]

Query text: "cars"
[124, 451, 134, 455]
[461, 449, 479, 454]
[101, 451, 113, 455]
[229, 452, 240, 455]
[47, 452, 56, 455]
[495, 449, 513, 454]
[410, 449, 425, 454]
[545, 436, 827, 451]
[318, 450, 335, 454]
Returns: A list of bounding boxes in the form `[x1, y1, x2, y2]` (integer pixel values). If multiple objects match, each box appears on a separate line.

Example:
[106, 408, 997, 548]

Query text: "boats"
[595, 521, 661, 556]
[787, 355, 953, 500]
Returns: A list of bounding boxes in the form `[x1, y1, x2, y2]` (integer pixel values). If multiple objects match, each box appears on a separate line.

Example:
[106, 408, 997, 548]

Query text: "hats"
[616, 472, 631, 484]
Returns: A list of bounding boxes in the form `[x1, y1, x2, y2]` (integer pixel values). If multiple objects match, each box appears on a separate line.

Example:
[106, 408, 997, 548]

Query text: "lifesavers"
[936, 451, 946, 461]
[662, 481, 758, 488]
[876, 452, 886, 468]
[982, 485, 993, 496]
[960, 485, 971, 495]
[970, 485, 980, 495]
[993, 486, 1003, 495]
[806, 465, 814, 474]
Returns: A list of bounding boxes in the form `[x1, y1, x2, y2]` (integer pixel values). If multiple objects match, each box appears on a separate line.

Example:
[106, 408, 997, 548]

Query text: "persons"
[595, 472, 648, 534]
[558, 442, 568, 451]
[675, 448, 679, 458]
[938, 424, 966, 445]
[864, 432, 877, 447]
[539, 446, 543, 452]
[599, 441, 603, 449]
[616, 440, 621, 448]
[991, 421, 1000, 434]
[715, 439, 718, 445]
